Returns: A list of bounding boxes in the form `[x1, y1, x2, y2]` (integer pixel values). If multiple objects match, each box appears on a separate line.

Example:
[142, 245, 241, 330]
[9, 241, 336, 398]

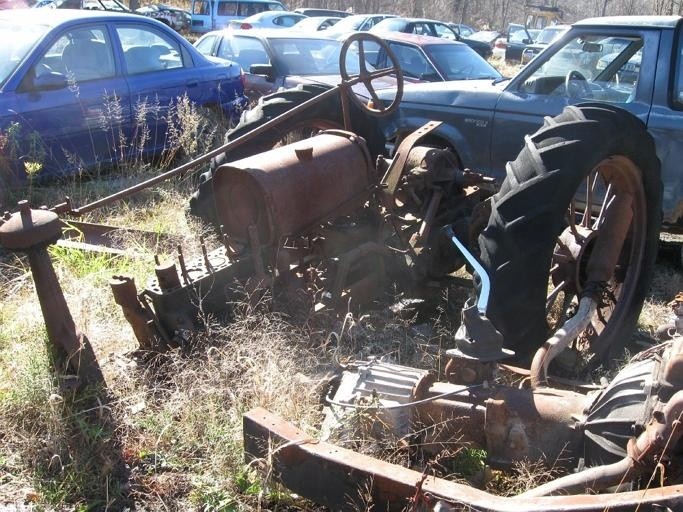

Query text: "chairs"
[15, 36, 166, 83]
[235, 48, 318, 74]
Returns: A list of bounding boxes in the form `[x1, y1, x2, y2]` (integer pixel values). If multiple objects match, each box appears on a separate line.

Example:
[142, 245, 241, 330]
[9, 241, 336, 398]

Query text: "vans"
[192, 0, 288, 34]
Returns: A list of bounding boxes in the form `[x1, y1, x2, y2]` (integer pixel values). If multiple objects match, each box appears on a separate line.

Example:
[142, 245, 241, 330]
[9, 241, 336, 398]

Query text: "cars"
[0, 5, 246, 188]
